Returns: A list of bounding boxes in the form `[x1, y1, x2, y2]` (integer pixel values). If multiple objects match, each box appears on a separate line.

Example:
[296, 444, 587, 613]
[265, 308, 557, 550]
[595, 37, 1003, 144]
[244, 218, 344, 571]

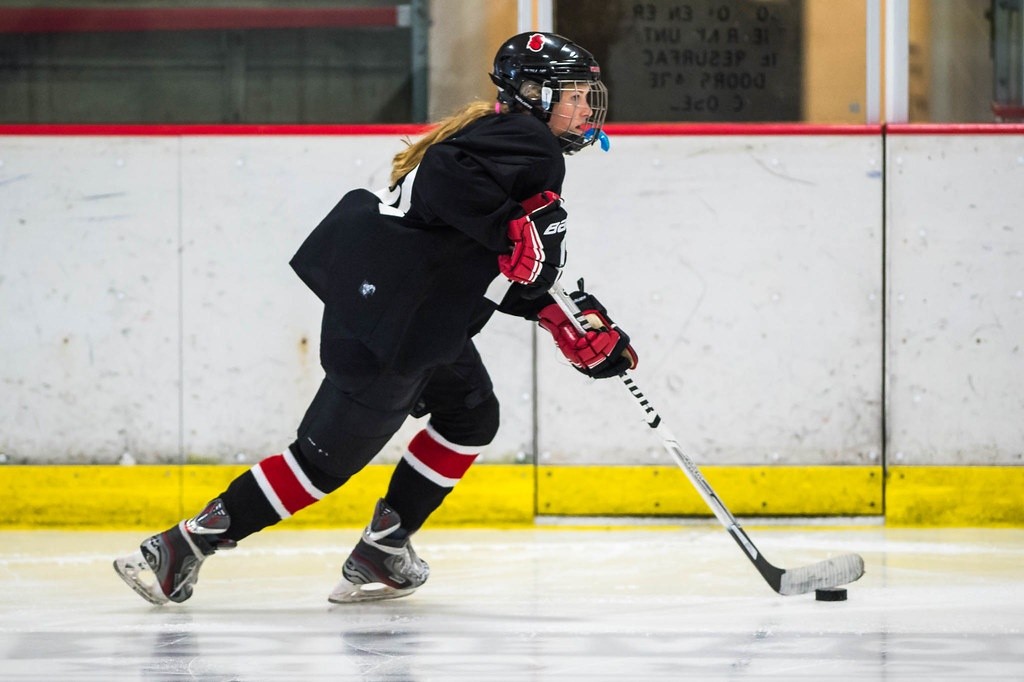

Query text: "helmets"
[489, 31, 608, 153]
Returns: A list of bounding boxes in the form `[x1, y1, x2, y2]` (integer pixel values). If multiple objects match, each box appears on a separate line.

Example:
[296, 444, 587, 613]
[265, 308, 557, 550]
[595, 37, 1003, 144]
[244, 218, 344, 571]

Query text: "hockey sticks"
[549, 288, 865, 596]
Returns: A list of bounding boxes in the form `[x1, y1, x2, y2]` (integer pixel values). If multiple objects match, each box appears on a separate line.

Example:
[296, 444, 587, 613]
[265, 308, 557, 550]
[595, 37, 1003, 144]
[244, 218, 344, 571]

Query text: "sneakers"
[329, 498, 429, 604]
[114, 498, 238, 607]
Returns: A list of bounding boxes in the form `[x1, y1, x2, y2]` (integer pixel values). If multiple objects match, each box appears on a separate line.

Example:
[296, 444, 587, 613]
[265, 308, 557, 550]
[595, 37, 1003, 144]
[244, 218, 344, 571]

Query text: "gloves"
[538, 276, 641, 379]
[497, 191, 567, 298]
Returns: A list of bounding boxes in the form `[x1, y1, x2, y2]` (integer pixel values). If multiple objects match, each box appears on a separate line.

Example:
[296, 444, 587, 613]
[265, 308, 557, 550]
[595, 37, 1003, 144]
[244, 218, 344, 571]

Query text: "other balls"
[816, 588, 849, 603]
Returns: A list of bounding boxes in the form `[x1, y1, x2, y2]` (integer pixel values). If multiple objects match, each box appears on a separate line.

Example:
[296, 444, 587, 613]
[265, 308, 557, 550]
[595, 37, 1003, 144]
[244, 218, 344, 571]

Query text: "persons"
[114, 32, 638, 605]
